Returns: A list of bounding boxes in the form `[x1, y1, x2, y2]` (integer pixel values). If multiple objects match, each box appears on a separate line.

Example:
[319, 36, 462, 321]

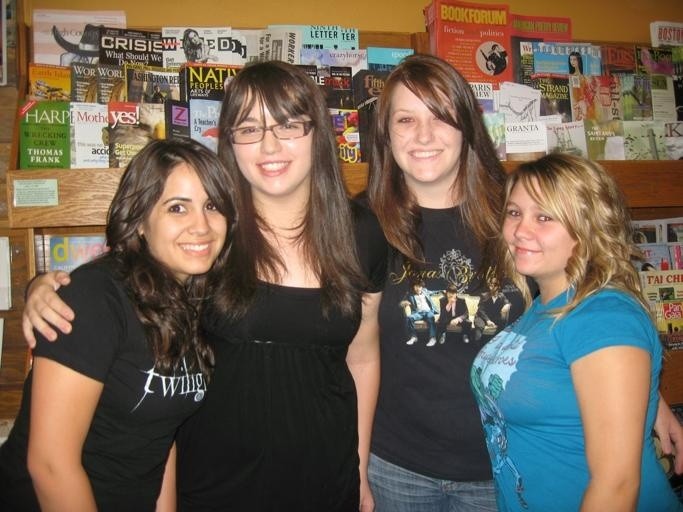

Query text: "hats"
[51, 25, 104, 59]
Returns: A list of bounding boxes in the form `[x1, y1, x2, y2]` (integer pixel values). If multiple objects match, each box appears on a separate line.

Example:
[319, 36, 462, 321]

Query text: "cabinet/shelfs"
[3, 0, 683, 427]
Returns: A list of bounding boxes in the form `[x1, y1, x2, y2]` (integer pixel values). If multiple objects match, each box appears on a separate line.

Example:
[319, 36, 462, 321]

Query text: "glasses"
[226, 121, 314, 144]
